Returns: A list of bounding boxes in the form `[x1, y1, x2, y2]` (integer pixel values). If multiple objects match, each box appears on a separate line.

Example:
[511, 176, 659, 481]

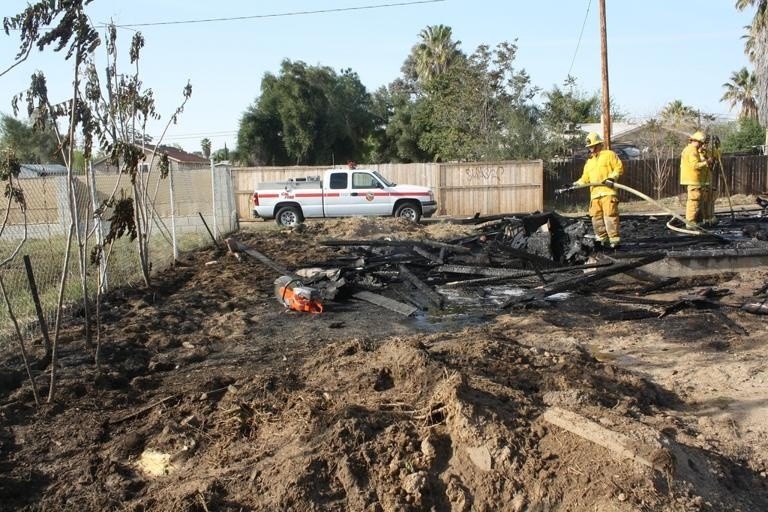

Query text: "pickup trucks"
[252, 161, 437, 229]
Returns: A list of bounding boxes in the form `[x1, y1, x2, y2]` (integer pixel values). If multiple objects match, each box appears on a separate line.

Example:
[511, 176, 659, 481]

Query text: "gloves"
[601, 177, 615, 188]
[562, 183, 573, 193]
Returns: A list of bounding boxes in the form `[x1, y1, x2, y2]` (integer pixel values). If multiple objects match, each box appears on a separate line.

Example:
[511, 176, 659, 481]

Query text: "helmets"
[688, 132, 708, 144]
[585, 131, 604, 148]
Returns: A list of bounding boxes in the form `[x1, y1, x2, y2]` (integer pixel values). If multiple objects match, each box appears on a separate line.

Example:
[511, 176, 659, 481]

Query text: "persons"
[678, 131, 708, 231]
[698, 134, 720, 224]
[557, 130, 624, 251]
